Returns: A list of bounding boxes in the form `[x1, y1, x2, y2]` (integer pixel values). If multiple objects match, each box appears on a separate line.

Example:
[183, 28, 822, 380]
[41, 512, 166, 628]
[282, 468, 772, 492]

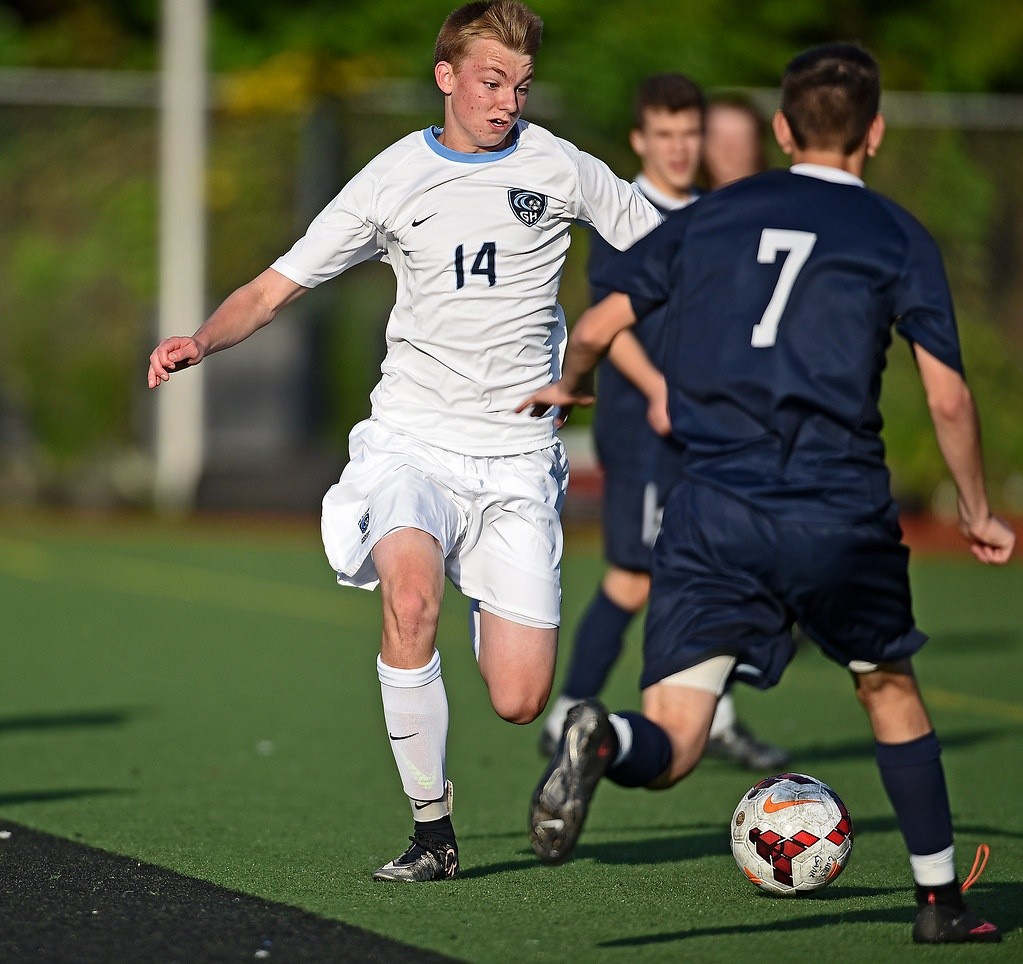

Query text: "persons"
[514, 44, 1013, 941]
[697, 92, 785, 771]
[148, 0, 665, 885]
[538, 75, 712, 760]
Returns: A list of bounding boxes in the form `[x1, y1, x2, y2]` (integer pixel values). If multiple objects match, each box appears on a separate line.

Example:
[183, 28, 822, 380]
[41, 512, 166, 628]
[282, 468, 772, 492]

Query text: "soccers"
[729, 773, 853, 897]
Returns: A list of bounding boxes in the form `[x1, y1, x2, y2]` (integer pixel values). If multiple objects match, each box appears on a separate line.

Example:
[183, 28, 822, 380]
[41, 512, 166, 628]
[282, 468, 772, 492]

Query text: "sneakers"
[537, 694, 582, 754]
[914, 844, 1002, 943]
[701, 717, 789, 773]
[527, 701, 621, 866]
[371, 833, 459, 882]
